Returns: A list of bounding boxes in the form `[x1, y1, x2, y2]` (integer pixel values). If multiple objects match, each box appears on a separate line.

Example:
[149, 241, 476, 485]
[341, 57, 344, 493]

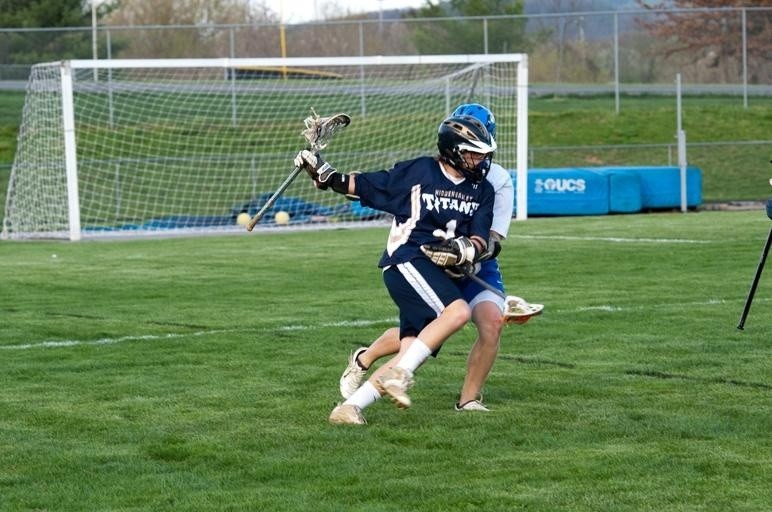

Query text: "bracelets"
[331, 173, 349, 194]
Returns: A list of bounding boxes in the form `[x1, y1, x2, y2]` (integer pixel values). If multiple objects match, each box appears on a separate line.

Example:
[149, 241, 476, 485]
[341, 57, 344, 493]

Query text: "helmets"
[437, 115, 497, 185]
[451, 103, 497, 142]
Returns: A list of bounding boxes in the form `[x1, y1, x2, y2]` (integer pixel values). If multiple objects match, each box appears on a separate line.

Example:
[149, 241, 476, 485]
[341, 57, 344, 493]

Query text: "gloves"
[420, 235, 477, 269]
[293, 147, 338, 190]
[444, 238, 502, 282]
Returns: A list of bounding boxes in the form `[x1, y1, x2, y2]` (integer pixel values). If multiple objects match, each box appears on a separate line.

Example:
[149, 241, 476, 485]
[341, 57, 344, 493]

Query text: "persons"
[339, 103, 514, 411]
[294, 115, 498, 426]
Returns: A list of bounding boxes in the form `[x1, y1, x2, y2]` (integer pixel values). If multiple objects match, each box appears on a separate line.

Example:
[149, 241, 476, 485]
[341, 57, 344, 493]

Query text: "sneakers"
[455, 399, 497, 411]
[340, 347, 370, 400]
[329, 402, 368, 425]
[373, 366, 416, 410]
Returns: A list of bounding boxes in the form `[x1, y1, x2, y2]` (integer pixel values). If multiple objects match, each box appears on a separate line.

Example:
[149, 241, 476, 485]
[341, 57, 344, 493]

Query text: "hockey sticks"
[467, 272, 545, 325]
[247, 106, 351, 231]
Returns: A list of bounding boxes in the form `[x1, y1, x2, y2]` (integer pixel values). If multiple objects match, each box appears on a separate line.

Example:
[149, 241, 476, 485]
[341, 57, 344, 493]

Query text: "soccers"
[274, 211, 290, 223]
[236, 213, 251, 226]
